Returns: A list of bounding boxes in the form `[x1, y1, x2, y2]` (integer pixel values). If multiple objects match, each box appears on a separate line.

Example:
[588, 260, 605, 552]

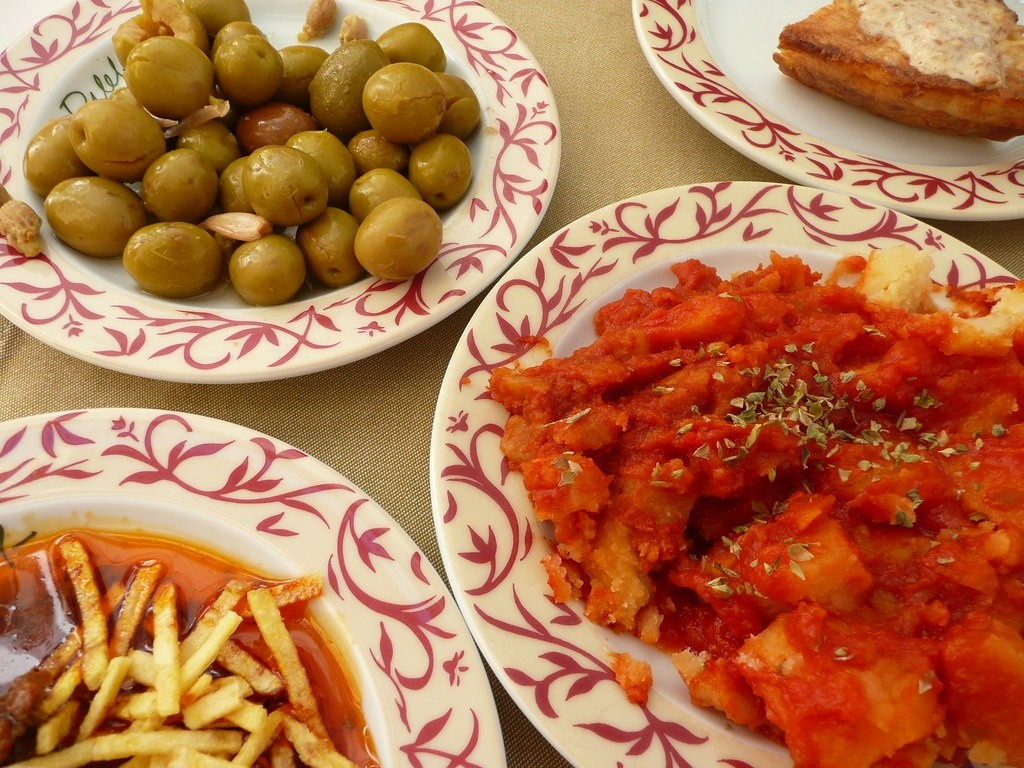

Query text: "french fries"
[0, 540, 366, 768]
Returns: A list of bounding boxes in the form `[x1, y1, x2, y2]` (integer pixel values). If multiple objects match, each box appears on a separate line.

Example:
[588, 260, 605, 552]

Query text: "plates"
[0, 0, 564, 386]
[427, 178, 1024, 768]
[630, 0, 1024, 222]
[0, 406, 507, 768]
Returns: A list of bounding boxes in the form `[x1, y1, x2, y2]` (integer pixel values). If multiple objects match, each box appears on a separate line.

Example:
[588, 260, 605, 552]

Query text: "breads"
[771, 0, 1024, 142]
